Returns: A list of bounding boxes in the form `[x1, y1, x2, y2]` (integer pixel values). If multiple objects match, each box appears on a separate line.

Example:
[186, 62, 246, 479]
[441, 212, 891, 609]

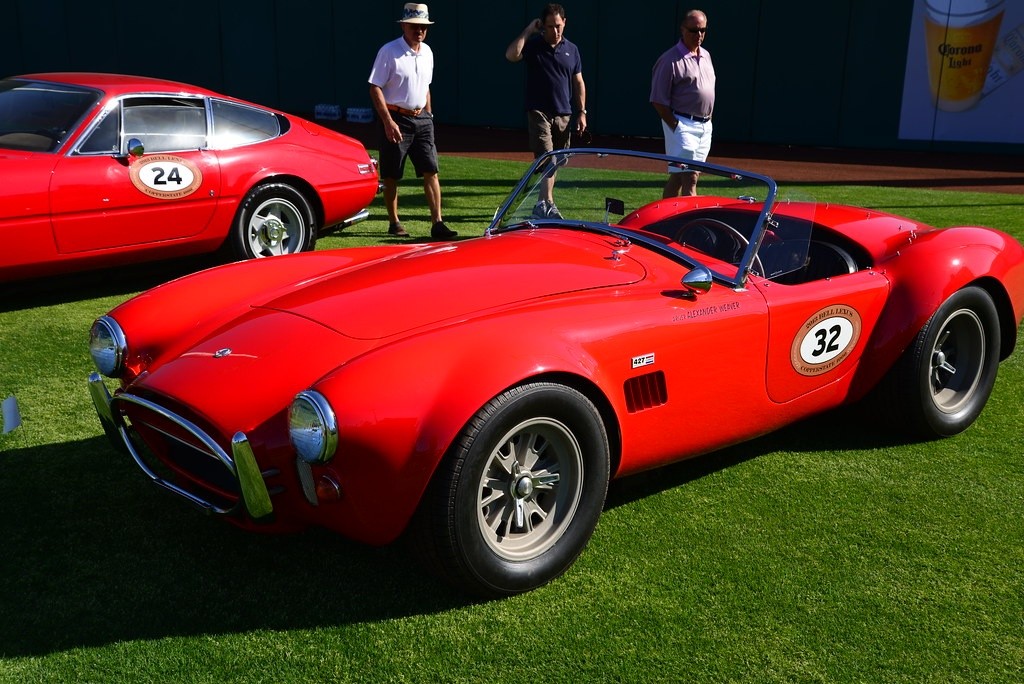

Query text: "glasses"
[683, 25, 706, 33]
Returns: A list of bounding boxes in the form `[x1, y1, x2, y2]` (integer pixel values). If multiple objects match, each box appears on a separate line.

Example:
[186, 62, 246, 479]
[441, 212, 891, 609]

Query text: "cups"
[923, 1, 1006, 114]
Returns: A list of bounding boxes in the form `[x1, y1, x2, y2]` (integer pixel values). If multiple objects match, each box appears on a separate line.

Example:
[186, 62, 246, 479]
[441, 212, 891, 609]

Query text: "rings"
[392, 139, 395, 141]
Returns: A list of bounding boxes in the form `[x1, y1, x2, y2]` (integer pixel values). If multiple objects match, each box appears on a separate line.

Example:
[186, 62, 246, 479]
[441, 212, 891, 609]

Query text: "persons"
[649, 10, 717, 200]
[368, 4, 458, 238]
[506, 4, 588, 220]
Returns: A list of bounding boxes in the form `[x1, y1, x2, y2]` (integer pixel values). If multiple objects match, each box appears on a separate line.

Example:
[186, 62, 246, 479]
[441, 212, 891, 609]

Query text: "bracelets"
[670, 118, 678, 128]
[578, 109, 587, 114]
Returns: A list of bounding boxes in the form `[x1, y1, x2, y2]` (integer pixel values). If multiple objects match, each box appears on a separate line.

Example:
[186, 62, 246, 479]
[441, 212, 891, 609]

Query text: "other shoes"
[430, 221, 458, 237]
[388, 223, 410, 237]
[533, 200, 547, 217]
[546, 199, 563, 219]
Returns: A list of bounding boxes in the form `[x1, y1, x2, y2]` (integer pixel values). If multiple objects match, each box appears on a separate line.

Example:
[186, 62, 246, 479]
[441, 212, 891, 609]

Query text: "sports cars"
[87, 148, 1024, 595]
[0, 72, 385, 283]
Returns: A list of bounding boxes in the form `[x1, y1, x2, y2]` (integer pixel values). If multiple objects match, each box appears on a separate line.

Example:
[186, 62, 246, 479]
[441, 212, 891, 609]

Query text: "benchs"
[645, 219, 858, 285]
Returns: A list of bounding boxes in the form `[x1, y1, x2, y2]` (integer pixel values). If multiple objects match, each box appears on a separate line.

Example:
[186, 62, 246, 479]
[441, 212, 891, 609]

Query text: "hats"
[397, 3, 435, 25]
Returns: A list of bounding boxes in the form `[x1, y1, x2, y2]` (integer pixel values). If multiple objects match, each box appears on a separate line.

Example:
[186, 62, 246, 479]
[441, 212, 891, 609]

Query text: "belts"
[387, 104, 422, 117]
[673, 111, 712, 125]
